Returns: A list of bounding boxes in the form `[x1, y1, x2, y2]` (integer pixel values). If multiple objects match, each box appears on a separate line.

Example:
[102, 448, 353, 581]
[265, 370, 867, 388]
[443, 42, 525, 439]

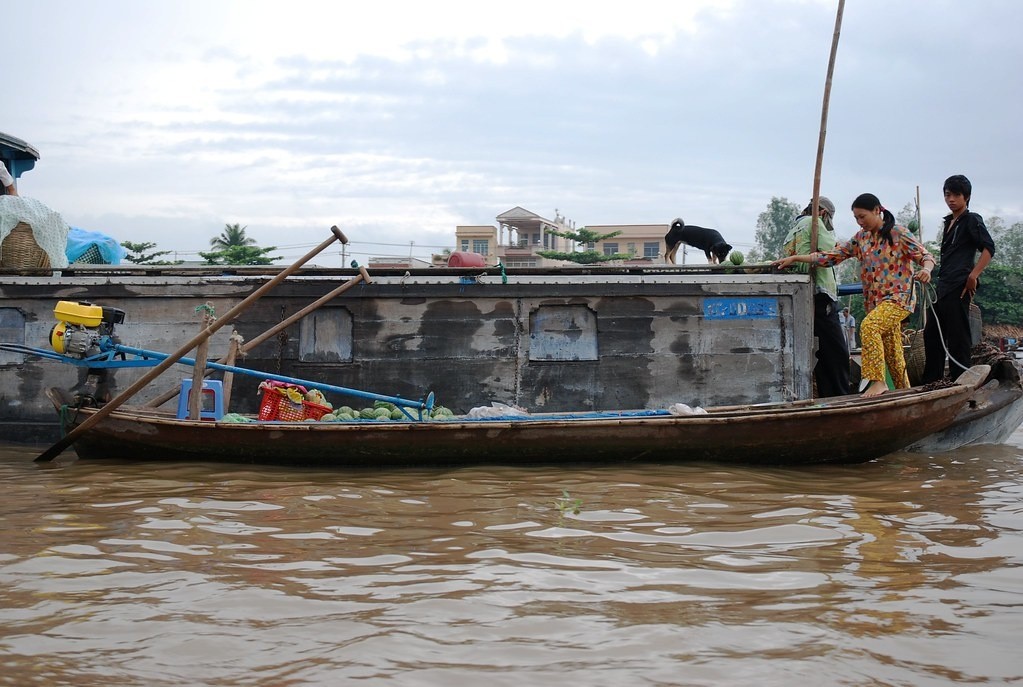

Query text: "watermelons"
[718, 250, 785, 274]
[321, 401, 455, 423]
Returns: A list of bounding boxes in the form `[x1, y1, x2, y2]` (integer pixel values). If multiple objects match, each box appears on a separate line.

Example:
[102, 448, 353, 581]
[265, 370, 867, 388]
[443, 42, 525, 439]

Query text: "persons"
[0, 161, 15, 196]
[770, 193, 935, 399]
[920, 175, 995, 383]
[842, 307, 856, 349]
[784, 197, 851, 395]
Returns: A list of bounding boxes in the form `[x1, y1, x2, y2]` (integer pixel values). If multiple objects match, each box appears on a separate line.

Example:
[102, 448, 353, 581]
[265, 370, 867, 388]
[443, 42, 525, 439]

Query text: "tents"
[838, 282, 862, 295]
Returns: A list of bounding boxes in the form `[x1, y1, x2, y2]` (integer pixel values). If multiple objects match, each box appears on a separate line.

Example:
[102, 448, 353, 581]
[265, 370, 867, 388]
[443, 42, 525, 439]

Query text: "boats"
[0, 267, 1023, 454]
[41, 297, 993, 469]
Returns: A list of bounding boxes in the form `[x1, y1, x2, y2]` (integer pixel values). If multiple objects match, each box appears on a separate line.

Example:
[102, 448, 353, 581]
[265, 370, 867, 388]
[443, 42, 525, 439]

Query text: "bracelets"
[924, 269, 930, 272]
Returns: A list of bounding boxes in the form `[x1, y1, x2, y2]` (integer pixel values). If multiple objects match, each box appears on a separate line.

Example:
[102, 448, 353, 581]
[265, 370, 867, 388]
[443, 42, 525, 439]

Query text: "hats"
[808, 196, 836, 232]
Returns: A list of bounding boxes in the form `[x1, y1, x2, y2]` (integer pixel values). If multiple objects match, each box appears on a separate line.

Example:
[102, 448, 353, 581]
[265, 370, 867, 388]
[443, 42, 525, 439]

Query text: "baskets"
[259, 386, 333, 423]
[1, 221, 67, 276]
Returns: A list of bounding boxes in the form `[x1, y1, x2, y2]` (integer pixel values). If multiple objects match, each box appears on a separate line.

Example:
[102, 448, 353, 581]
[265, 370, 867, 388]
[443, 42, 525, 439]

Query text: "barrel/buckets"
[447, 251, 485, 267]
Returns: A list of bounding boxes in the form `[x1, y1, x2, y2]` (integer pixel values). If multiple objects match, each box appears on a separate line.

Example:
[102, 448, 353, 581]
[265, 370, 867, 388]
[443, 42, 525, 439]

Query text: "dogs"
[664, 217, 732, 265]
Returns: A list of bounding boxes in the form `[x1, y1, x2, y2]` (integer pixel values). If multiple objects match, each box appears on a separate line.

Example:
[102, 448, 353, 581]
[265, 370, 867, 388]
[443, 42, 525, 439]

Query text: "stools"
[177, 380, 223, 421]
